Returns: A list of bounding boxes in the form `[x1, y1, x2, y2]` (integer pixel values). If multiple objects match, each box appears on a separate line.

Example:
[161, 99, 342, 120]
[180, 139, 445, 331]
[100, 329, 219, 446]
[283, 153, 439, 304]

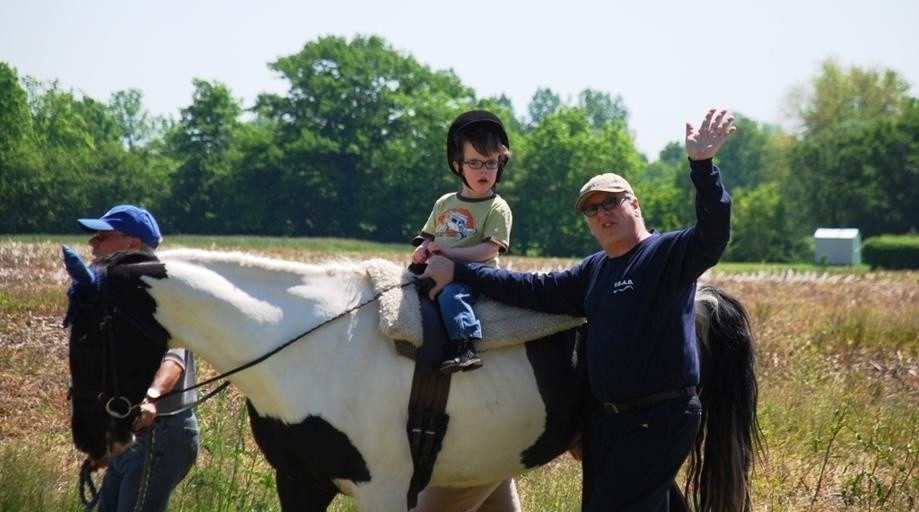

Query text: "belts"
[604, 387, 696, 416]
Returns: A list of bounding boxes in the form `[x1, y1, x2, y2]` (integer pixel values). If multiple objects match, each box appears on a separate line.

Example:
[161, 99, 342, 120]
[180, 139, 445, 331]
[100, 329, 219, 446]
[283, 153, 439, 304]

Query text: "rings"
[717, 130, 721, 135]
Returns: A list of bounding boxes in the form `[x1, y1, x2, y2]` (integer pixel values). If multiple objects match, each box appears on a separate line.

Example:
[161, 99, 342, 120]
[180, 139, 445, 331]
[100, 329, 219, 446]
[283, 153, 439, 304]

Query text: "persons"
[411, 111, 514, 376]
[77, 204, 200, 512]
[418, 109, 736, 512]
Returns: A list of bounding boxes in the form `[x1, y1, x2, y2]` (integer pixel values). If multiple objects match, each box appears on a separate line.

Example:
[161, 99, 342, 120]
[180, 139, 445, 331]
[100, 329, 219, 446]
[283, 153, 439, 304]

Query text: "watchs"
[146, 388, 161, 400]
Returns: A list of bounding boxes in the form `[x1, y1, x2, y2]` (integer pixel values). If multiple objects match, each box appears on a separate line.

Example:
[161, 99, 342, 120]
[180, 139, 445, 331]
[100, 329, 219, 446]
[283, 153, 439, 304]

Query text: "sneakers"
[440, 339, 483, 372]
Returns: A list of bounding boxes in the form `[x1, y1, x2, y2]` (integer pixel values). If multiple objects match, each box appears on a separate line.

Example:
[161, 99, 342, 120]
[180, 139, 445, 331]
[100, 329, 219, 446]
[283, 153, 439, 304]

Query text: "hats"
[447, 110, 510, 174]
[575, 172, 634, 210]
[77, 204, 160, 248]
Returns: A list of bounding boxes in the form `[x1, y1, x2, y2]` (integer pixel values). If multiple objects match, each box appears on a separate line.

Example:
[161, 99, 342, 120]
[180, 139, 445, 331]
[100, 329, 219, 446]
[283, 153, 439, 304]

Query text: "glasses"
[583, 196, 617, 216]
[462, 159, 497, 169]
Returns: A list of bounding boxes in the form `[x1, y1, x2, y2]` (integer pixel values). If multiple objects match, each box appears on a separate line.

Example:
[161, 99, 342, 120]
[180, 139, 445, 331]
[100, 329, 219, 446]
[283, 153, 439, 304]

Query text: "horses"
[62, 244, 770, 512]
